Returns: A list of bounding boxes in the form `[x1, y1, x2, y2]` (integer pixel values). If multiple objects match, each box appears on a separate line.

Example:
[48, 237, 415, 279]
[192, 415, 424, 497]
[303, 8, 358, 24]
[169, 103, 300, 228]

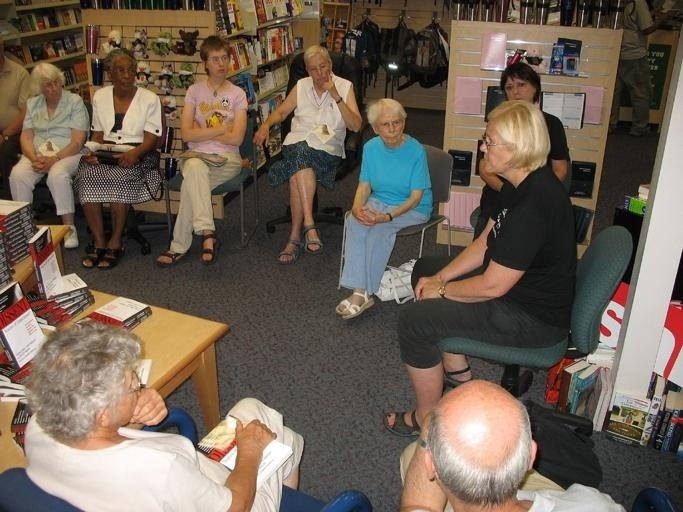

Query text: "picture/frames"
[0, 224, 69, 293]
[0, 282, 229, 470]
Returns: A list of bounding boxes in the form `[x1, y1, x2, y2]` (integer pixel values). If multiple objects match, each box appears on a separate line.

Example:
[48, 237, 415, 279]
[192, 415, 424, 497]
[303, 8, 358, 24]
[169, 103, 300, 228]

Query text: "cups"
[481, 134, 510, 149]
[209, 55, 230, 64]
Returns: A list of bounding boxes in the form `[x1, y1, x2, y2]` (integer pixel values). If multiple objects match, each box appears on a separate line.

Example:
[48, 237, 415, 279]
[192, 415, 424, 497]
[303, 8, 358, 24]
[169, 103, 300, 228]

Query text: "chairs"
[64, 225, 79, 249]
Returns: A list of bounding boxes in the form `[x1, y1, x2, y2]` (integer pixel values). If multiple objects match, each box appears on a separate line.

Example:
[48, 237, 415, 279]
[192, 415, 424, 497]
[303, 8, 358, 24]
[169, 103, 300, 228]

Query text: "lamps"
[165, 119, 258, 247]
[85, 127, 172, 256]
[0, 406, 372, 511]
[6, 103, 91, 221]
[438, 227, 633, 439]
[469, 150, 571, 228]
[262, 51, 364, 234]
[337, 144, 452, 302]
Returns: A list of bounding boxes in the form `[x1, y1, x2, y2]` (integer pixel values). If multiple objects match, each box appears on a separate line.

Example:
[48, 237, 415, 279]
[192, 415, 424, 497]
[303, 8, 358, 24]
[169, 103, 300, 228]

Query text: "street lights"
[99, 148, 164, 202]
[374, 259, 418, 305]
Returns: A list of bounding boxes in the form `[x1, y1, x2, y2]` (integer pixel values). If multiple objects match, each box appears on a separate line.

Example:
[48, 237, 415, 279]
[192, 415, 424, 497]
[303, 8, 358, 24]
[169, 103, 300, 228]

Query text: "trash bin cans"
[2, 133, 9, 144]
[438, 281, 447, 300]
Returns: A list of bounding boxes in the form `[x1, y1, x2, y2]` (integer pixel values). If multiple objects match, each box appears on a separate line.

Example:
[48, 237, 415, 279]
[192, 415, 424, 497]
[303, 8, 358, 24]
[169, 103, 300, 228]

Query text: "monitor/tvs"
[0, 282, 49, 371]
[135, 358, 153, 388]
[549, 37, 583, 76]
[622, 183, 651, 216]
[454, 77, 481, 114]
[194, 414, 295, 491]
[0, 9, 90, 105]
[258, 89, 286, 131]
[11, 403, 32, 457]
[29, 226, 63, 300]
[582, 86, 605, 124]
[232, 62, 290, 105]
[542, 92, 586, 129]
[75, 296, 153, 333]
[258, 132, 281, 167]
[443, 189, 482, 229]
[485, 86, 506, 121]
[225, 24, 303, 72]
[571, 161, 596, 196]
[447, 149, 473, 186]
[0, 198, 38, 293]
[216, 0, 303, 35]
[0, 359, 34, 403]
[543, 359, 610, 431]
[481, 31, 506, 70]
[639, 376, 683, 456]
[26, 272, 96, 332]
[605, 393, 650, 447]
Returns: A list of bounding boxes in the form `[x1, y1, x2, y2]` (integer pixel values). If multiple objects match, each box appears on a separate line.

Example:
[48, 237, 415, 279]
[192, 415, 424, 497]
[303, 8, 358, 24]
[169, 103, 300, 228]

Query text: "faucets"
[383, 410, 422, 437]
[335, 293, 351, 315]
[82, 247, 104, 269]
[156, 250, 187, 266]
[96, 247, 125, 271]
[200, 233, 217, 265]
[276, 239, 302, 266]
[342, 292, 374, 320]
[444, 366, 472, 388]
[303, 225, 324, 255]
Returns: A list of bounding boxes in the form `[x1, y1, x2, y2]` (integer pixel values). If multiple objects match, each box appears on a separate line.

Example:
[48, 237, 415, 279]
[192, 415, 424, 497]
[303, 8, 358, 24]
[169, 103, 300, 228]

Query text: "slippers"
[342, 18, 380, 97]
[380, 20, 416, 98]
[396, 23, 450, 92]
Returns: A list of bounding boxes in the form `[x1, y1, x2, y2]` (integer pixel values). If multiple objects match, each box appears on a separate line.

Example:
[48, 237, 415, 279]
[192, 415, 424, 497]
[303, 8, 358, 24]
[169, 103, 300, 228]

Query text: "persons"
[608, 0, 668, 138]
[24, 326, 304, 512]
[0, 35, 90, 248]
[473, 64, 570, 242]
[398, 378, 627, 511]
[253, 44, 363, 265]
[73, 49, 163, 271]
[335, 98, 433, 321]
[154, 36, 248, 267]
[382, 101, 574, 438]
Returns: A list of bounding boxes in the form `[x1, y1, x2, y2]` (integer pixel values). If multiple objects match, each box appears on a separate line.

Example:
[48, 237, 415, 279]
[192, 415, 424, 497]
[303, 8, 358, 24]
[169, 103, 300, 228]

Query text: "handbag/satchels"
[386, 213, 392, 222]
[336, 98, 342, 103]
